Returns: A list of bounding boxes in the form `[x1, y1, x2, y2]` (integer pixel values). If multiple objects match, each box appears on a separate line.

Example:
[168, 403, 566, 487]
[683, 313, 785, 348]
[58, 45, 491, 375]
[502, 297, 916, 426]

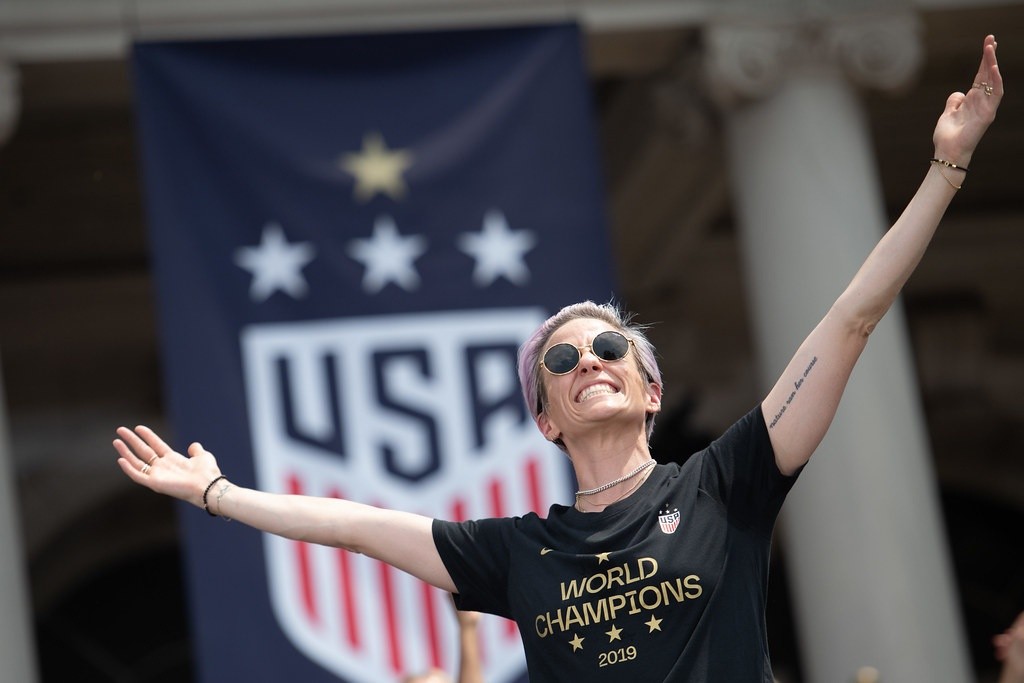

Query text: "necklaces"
[575, 459, 657, 512]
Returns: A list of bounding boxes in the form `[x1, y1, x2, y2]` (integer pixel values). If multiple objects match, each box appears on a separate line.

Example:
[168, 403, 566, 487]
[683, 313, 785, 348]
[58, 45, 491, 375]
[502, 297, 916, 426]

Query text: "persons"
[111, 33, 1004, 683]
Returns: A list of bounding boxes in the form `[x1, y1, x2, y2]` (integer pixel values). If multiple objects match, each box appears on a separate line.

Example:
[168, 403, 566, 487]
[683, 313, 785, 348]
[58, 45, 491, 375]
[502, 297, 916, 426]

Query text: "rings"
[985, 87, 993, 95]
[981, 82, 987, 86]
[141, 464, 150, 473]
[148, 455, 158, 465]
[972, 83, 980, 88]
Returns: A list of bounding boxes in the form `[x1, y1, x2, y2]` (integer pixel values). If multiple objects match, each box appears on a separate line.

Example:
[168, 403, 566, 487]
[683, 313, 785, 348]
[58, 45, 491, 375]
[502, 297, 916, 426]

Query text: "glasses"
[538, 331, 635, 375]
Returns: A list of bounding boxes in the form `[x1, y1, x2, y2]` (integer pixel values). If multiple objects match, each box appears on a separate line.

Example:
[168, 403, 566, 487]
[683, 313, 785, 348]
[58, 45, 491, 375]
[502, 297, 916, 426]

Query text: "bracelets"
[203, 475, 226, 516]
[931, 161, 961, 189]
[216, 485, 232, 521]
[931, 158, 970, 172]
